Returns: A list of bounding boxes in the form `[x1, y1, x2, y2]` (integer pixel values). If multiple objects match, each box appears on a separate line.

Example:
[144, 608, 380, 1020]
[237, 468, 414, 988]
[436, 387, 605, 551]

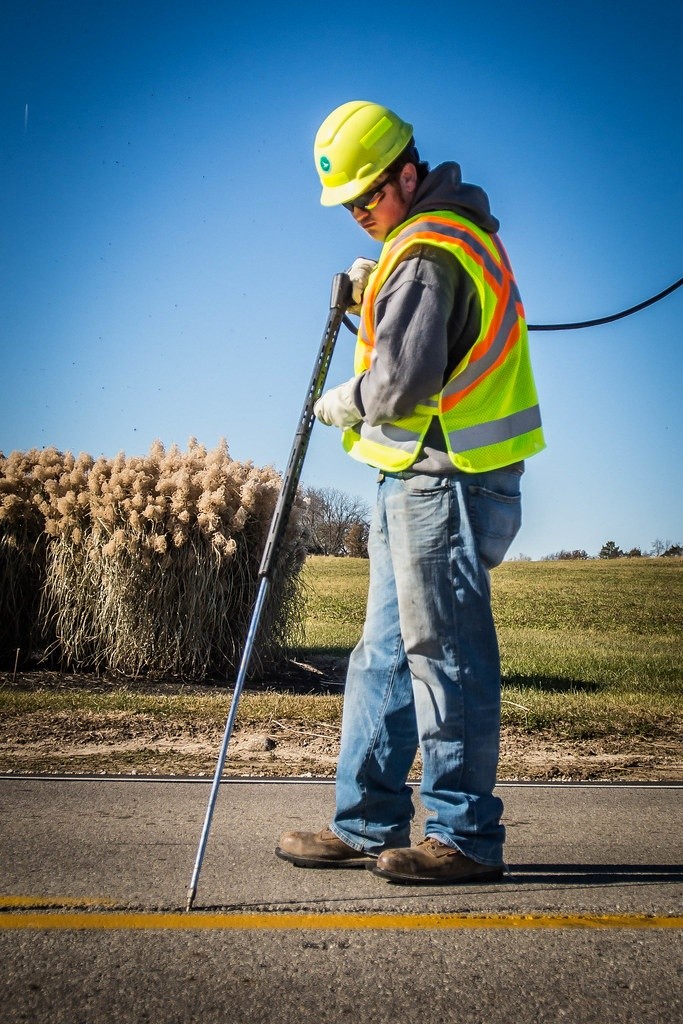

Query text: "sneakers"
[376, 837, 498, 883]
[275, 825, 377, 870]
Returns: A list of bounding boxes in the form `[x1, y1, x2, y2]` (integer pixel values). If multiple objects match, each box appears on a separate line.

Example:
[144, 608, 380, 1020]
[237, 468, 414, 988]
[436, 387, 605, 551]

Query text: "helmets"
[313, 100, 413, 206]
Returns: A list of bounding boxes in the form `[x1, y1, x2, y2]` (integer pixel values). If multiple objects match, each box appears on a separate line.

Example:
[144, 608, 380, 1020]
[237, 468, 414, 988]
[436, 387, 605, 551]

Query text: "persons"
[272, 100, 548, 886]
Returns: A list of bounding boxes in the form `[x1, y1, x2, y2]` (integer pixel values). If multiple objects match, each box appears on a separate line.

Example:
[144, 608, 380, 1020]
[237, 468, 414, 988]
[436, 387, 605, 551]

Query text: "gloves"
[347, 257, 377, 304]
[312, 370, 366, 431]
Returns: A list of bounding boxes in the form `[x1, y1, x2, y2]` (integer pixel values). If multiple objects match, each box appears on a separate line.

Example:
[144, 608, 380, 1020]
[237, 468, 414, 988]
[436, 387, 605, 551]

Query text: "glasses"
[342, 168, 400, 212]
